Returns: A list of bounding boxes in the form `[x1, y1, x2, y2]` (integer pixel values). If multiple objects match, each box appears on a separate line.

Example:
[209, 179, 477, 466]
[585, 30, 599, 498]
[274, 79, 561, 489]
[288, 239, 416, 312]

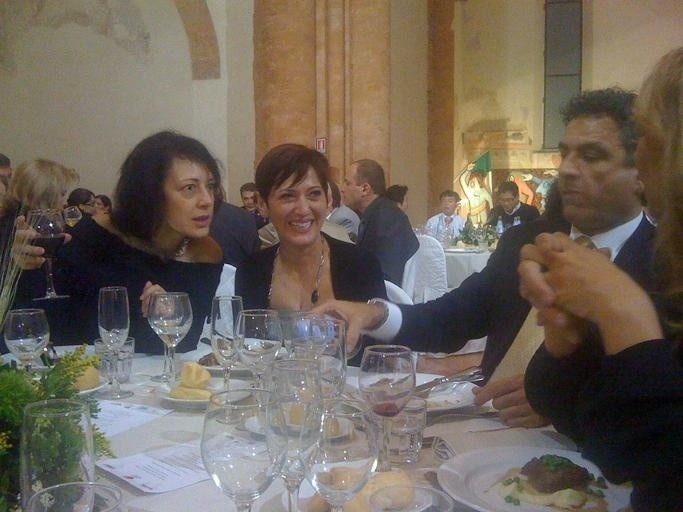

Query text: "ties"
[484, 235, 595, 386]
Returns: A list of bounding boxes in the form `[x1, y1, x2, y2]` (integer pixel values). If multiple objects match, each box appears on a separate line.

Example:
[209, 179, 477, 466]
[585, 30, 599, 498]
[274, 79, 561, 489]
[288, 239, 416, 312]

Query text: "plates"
[444, 248, 478, 252]
[436, 444, 631, 512]
[347, 366, 490, 418]
[26, 368, 112, 399]
[181, 336, 289, 381]
[151, 376, 250, 408]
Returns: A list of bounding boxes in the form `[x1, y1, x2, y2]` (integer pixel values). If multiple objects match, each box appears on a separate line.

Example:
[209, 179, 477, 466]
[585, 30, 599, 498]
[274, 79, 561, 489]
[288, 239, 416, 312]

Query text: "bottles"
[486, 215, 521, 251]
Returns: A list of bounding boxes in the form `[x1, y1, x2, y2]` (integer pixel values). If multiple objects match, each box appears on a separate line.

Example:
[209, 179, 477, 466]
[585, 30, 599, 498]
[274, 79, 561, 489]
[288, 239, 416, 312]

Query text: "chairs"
[410, 236, 449, 303]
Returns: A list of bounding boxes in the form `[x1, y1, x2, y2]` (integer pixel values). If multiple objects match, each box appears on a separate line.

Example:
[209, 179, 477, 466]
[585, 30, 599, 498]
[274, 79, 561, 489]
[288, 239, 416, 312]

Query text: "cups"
[22, 402, 125, 512]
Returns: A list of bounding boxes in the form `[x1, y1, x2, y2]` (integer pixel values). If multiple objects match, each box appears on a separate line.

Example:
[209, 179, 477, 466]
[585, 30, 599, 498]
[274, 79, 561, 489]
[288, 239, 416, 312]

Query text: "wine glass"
[414, 221, 453, 248]
[145, 293, 195, 385]
[359, 346, 420, 474]
[2, 307, 49, 381]
[92, 286, 135, 400]
[203, 295, 374, 512]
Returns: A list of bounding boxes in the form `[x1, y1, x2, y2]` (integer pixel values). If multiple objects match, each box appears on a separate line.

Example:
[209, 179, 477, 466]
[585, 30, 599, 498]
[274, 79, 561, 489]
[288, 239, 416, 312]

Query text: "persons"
[486, 178, 540, 232]
[302, 86, 656, 425]
[340, 159, 422, 289]
[1, 130, 257, 356]
[421, 190, 465, 244]
[234, 140, 388, 370]
[513, 48, 683, 511]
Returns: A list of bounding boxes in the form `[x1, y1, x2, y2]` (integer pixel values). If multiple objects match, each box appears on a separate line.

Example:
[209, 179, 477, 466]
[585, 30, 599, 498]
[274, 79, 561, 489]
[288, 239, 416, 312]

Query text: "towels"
[180, 264, 237, 360]
